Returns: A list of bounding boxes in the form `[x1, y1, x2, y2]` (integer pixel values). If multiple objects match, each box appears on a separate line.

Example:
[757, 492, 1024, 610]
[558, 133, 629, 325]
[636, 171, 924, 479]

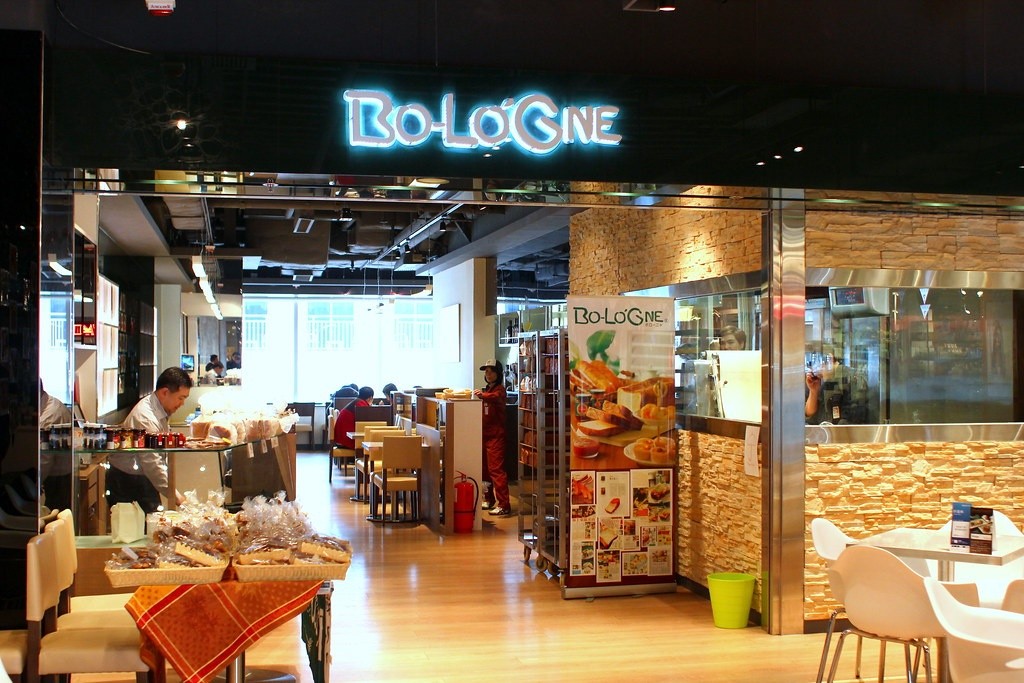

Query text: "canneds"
[39, 423, 71, 450]
[82, 423, 147, 450]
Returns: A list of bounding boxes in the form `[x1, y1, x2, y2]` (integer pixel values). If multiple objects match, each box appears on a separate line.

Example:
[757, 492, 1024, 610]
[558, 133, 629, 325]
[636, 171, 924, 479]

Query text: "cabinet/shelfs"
[517, 328, 571, 588]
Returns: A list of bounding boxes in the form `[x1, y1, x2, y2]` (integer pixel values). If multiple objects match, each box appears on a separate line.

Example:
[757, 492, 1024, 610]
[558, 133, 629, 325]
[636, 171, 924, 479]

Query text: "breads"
[191, 418, 284, 443]
[519, 338, 570, 467]
[573, 359, 675, 437]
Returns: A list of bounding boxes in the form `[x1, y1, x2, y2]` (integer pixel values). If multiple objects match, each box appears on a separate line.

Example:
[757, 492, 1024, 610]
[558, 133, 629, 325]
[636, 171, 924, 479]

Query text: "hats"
[479, 359, 503, 372]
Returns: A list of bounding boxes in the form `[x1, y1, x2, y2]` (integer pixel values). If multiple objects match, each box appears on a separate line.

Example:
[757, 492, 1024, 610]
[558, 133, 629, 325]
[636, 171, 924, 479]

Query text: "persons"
[804, 340, 870, 426]
[227, 352, 242, 370]
[473, 359, 511, 515]
[39, 377, 72, 512]
[206, 355, 222, 378]
[383, 383, 398, 401]
[206, 361, 224, 378]
[335, 386, 374, 449]
[326, 384, 358, 417]
[718, 326, 746, 350]
[105, 367, 192, 535]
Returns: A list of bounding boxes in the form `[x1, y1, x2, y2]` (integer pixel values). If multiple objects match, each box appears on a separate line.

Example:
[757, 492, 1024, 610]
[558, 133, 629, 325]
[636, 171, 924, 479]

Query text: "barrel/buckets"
[707, 572, 755, 629]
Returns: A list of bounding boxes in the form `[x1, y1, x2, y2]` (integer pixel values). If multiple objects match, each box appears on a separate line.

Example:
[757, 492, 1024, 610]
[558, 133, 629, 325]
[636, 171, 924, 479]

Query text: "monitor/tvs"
[181, 355, 194, 372]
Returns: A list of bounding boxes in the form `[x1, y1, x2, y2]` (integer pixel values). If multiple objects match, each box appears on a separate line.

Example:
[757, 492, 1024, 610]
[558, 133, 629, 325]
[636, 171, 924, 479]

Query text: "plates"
[624, 442, 676, 468]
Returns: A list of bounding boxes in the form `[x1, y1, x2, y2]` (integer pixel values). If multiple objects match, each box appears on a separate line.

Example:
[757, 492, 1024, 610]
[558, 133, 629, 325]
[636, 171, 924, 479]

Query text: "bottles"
[505, 317, 519, 343]
[194, 408, 202, 419]
[264, 403, 277, 434]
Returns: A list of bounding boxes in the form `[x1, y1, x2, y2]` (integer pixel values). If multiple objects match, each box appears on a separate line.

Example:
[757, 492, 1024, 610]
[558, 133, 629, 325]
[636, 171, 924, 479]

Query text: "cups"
[632, 410, 674, 426]
[573, 389, 606, 459]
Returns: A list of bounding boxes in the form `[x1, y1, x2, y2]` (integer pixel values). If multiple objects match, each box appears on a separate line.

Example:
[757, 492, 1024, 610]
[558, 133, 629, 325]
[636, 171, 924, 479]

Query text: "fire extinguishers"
[454, 470, 478, 533]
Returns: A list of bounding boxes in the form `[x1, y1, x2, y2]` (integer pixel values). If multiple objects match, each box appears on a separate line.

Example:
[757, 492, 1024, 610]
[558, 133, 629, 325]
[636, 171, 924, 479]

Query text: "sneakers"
[489, 507, 509, 514]
[481, 501, 493, 509]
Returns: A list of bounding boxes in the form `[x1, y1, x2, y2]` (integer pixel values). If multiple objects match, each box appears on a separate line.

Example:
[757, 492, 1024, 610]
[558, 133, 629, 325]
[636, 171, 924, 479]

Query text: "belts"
[107, 461, 142, 478]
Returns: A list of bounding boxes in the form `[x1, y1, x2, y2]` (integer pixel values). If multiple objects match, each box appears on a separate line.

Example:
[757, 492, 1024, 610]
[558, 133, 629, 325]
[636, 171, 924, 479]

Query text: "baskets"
[234, 549, 350, 582]
[105, 559, 229, 586]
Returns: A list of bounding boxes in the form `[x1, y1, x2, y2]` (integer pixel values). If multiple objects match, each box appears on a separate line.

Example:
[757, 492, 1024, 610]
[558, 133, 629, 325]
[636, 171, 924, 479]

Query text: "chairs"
[811, 509, 1024, 683]
[288, 402, 315, 451]
[24, 508, 156, 683]
[328, 407, 355, 483]
[354, 421, 422, 527]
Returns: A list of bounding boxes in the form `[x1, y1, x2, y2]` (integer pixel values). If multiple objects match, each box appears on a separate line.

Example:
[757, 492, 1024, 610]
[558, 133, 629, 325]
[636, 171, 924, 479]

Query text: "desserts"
[632, 403, 675, 463]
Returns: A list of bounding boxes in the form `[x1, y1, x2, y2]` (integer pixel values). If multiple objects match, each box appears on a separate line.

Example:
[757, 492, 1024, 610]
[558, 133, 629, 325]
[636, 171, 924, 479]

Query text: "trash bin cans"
[707, 573, 756, 629]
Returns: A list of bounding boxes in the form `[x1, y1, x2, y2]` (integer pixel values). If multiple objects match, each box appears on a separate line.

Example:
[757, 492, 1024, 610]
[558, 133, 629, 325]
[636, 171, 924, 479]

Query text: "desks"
[124, 579, 334, 683]
[347, 432, 429, 521]
[845, 528, 1024, 683]
